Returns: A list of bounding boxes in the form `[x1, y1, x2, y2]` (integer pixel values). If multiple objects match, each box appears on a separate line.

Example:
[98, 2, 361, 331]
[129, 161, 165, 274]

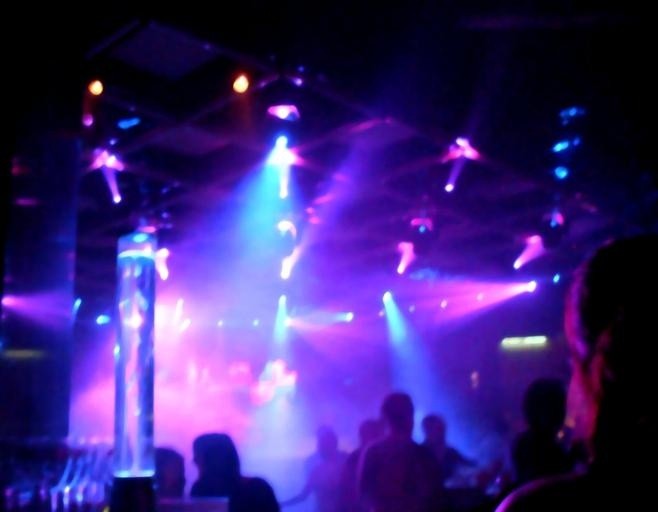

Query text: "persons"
[508, 377, 579, 483]
[494, 231, 656, 512]
[189, 432, 277, 512]
[277, 393, 476, 510]
[156, 447, 185, 501]
